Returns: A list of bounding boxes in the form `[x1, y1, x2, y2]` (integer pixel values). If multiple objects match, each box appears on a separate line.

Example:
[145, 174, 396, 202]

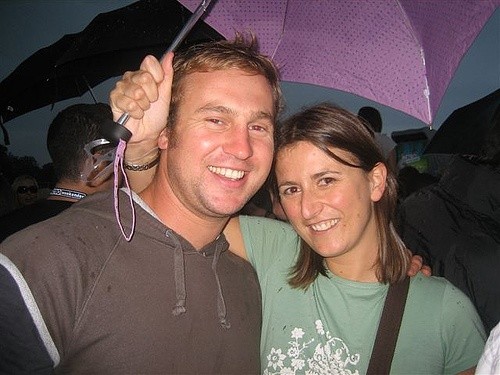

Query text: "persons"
[358, 106, 500, 375]
[0, 103, 123, 242]
[109, 51, 487, 374]
[0, 32, 432, 375]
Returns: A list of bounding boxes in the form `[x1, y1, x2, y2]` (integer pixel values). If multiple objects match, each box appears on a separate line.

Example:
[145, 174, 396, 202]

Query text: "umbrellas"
[0, 0, 500, 242]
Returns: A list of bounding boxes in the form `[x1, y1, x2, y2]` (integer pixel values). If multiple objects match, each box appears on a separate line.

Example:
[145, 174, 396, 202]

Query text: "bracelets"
[119, 149, 161, 172]
